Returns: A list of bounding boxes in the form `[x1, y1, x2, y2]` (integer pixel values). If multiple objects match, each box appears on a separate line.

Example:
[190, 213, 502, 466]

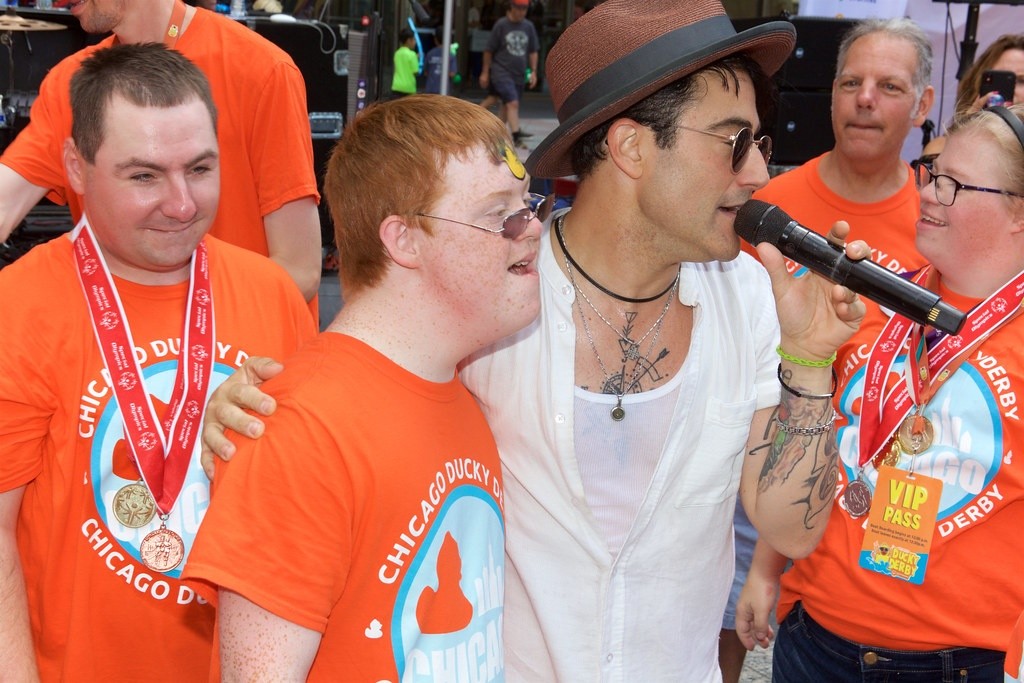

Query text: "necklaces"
[554, 211, 682, 422]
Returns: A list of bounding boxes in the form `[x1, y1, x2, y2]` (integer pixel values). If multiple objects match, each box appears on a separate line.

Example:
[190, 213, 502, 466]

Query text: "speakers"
[727, 14, 859, 166]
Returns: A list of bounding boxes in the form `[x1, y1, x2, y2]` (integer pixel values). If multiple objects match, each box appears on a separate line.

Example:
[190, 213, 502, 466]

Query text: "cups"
[37, 0, 52, 10]
[231, 0, 246, 17]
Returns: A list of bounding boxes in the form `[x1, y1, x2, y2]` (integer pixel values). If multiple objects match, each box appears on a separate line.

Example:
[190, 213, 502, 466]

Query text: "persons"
[720, 17, 932, 682]
[215, 89, 554, 683]
[390, 0, 597, 142]
[197, 0, 873, 681]
[0, 41, 317, 683]
[726, 103, 1024, 683]
[0, 0, 325, 338]
[958, 33, 1024, 115]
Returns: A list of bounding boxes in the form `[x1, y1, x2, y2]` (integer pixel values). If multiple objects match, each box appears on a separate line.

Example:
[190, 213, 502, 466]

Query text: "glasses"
[915, 163, 1024, 207]
[415, 193, 558, 239]
[605, 117, 773, 175]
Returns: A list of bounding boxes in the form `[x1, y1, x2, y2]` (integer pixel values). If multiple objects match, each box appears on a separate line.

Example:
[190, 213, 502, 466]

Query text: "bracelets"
[775, 408, 838, 434]
[777, 363, 838, 399]
[776, 343, 838, 367]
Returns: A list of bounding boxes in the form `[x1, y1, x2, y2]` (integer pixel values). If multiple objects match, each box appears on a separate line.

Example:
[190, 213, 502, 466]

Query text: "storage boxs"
[729, 16, 860, 90]
[227, 15, 349, 130]
[753, 90, 836, 166]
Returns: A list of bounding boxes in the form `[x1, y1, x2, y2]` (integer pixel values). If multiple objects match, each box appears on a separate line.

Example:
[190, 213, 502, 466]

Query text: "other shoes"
[512, 127, 534, 143]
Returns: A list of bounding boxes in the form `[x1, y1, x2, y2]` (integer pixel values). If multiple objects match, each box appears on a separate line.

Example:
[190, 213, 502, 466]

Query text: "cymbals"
[0, 14, 68, 30]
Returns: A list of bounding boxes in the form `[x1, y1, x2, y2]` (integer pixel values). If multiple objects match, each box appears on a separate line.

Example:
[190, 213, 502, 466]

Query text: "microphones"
[734, 198, 967, 336]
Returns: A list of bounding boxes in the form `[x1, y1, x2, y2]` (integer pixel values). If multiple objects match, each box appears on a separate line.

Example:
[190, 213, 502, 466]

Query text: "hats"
[524, 0, 797, 180]
[512, 0, 529, 9]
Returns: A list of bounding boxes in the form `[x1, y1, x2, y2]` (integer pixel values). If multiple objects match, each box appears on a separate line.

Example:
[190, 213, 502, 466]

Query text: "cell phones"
[980, 70, 1016, 111]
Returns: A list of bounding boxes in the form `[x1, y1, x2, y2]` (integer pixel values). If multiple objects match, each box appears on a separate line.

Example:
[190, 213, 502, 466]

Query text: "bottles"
[0, 95, 6, 127]
